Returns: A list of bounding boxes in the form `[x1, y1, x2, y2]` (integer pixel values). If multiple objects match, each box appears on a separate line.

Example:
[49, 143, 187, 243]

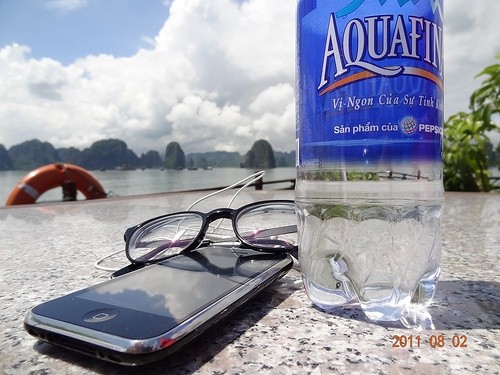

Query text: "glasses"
[108, 200, 299, 278]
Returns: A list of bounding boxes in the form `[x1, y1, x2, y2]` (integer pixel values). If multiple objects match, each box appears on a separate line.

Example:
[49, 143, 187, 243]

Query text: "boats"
[5, 162, 107, 206]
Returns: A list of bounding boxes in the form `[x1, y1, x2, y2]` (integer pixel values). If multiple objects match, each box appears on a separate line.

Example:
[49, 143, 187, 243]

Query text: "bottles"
[296, 1, 444, 321]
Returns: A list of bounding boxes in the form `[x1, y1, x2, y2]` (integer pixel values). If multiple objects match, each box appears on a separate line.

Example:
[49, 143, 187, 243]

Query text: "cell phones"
[25, 245, 293, 367]
